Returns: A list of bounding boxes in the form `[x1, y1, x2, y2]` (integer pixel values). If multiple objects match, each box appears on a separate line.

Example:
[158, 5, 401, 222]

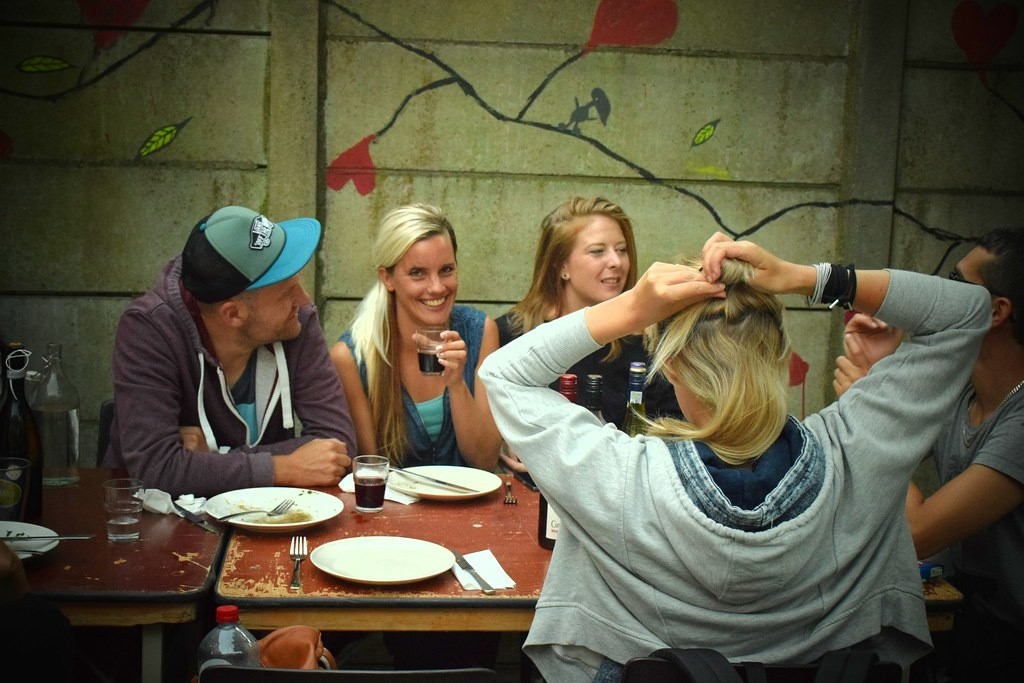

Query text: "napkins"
[452, 549, 518, 592]
[337, 471, 421, 506]
[133, 487, 207, 518]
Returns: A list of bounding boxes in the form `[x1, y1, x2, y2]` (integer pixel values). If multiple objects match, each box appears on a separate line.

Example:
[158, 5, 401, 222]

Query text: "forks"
[503, 481, 518, 505]
[291, 536, 309, 589]
[220, 500, 294, 527]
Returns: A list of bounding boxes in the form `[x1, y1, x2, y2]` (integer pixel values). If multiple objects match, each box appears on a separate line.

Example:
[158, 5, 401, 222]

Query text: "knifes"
[390, 466, 479, 493]
[173, 502, 222, 534]
[450, 549, 496, 595]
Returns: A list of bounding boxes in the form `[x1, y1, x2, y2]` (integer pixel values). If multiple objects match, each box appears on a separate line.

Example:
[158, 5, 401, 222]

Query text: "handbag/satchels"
[254, 624, 337, 670]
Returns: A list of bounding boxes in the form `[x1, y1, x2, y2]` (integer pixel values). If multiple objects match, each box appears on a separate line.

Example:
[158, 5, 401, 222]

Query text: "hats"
[181, 205, 321, 304]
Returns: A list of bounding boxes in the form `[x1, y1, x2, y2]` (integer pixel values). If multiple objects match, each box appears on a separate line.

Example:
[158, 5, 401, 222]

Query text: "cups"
[0, 457, 33, 521]
[353, 455, 389, 513]
[416, 326, 448, 376]
[102, 478, 144, 544]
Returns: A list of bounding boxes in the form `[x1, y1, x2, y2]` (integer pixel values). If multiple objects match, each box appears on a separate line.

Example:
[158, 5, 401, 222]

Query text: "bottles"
[197, 605, 261, 670]
[622, 362, 647, 436]
[538, 374, 607, 552]
[0, 341, 80, 524]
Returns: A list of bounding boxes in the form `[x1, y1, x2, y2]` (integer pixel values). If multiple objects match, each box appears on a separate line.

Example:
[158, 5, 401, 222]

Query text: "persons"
[832, 228, 1024, 683]
[494, 196, 684, 474]
[99, 206, 357, 498]
[331, 204, 502, 473]
[477, 230, 991, 683]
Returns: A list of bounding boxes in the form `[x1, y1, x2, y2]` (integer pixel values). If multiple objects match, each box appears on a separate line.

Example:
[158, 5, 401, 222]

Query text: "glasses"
[948, 272, 1017, 322]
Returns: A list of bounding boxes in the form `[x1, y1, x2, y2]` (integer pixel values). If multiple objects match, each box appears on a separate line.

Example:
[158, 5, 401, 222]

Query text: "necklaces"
[963, 382, 1024, 448]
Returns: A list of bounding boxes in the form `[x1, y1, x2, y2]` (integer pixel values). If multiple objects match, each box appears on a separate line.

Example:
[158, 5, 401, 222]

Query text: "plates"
[206, 487, 344, 534]
[387, 467, 502, 500]
[310, 536, 456, 585]
[0, 522, 60, 559]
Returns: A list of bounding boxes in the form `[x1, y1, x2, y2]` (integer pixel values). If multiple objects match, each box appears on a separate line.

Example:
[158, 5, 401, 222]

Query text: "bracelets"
[807, 262, 857, 312]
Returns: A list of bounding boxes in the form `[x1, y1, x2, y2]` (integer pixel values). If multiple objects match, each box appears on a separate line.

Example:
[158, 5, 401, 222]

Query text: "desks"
[216, 475, 963, 683]
[29, 468, 227, 683]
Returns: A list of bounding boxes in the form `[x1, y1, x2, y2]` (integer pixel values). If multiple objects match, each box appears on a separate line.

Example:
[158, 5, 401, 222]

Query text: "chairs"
[200, 665, 501, 683]
[621, 656, 903, 683]
[95, 400, 115, 468]
